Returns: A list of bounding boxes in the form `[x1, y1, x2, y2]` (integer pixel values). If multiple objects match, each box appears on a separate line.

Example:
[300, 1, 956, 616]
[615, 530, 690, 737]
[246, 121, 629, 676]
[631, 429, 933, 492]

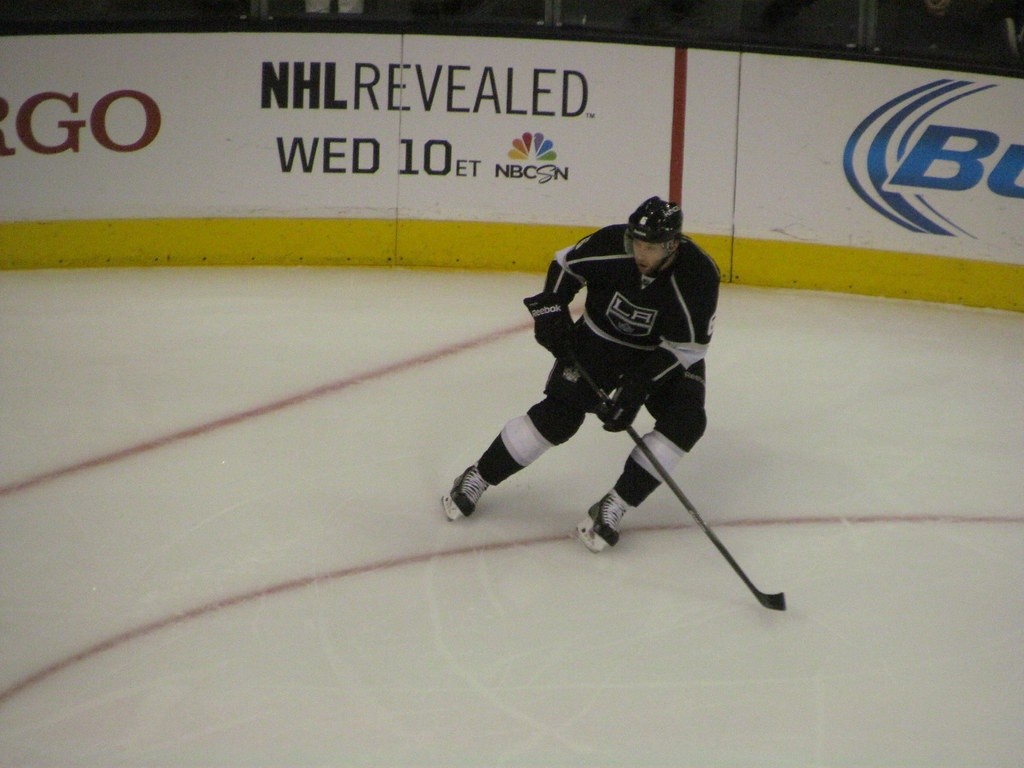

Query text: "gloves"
[595, 371, 649, 433]
[524, 291, 576, 359]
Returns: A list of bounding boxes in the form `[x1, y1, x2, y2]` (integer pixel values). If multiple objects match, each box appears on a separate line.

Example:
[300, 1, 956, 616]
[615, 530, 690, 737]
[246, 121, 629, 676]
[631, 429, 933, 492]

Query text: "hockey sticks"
[572, 360, 788, 612]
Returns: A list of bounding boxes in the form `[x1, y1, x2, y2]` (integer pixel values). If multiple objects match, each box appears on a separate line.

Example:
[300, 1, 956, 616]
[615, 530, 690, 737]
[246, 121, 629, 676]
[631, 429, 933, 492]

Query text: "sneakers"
[574, 488, 629, 553]
[441, 464, 490, 522]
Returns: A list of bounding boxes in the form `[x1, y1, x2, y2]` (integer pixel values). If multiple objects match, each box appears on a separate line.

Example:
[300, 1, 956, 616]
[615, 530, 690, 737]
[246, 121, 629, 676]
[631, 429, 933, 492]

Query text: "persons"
[442, 194, 720, 552]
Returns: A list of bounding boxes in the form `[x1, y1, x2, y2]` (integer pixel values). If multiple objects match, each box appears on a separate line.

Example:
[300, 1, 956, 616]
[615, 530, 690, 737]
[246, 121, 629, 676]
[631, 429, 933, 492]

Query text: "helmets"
[629, 196, 682, 243]
